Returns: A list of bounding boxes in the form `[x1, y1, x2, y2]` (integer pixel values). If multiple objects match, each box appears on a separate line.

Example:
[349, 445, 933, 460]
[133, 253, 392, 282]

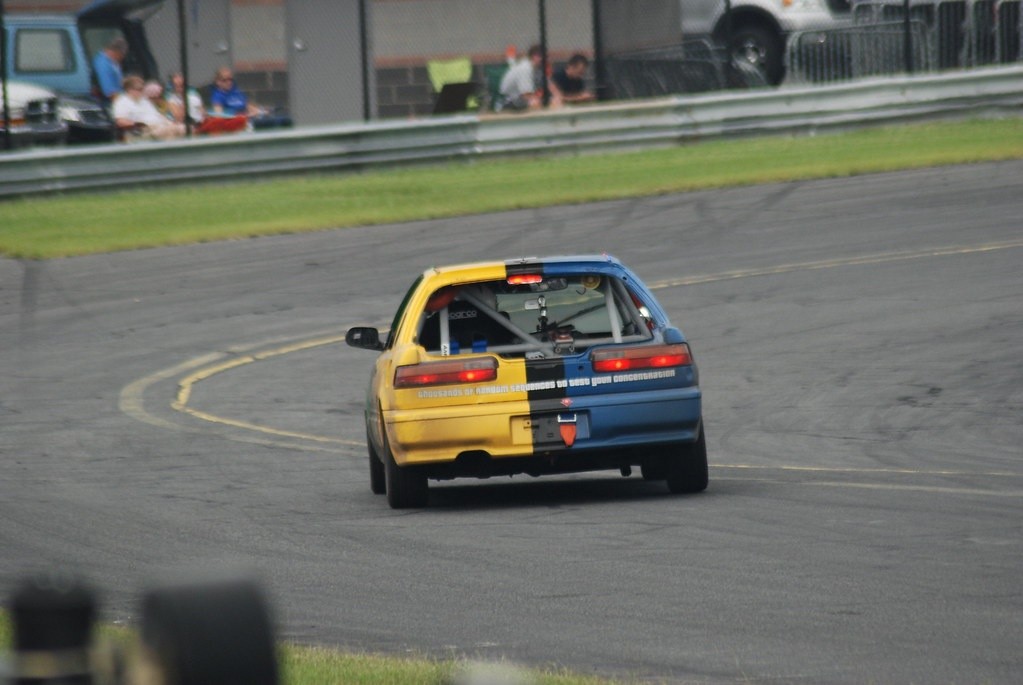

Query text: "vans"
[676, 0, 833, 87]
[2, 2, 165, 100]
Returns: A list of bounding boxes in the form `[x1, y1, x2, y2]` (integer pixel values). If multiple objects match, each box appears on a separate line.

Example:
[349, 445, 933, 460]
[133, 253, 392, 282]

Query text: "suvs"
[0, 83, 68, 148]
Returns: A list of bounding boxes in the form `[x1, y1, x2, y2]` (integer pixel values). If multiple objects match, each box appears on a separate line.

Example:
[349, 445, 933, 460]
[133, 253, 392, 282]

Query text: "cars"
[57, 95, 116, 143]
[345, 254, 710, 507]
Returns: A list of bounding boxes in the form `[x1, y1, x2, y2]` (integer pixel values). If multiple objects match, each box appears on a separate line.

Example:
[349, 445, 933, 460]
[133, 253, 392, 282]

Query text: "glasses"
[221, 78, 233, 82]
[133, 87, 143, 90]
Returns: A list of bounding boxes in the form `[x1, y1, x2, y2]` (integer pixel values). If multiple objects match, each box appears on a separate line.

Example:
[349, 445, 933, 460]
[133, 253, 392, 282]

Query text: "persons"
[169, 73, 206, 126]
[91, 36, 128, 101]
[111, 73, 187, 143]
[210, 66, 267, 117]
[494, 45, 561, 114]
[148, 76, 169, 114]
[545, 54, 594, 107]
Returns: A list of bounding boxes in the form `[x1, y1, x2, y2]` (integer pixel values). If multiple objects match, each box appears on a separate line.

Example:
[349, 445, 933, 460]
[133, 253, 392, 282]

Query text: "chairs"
[419, 300, 512, 351]
[103, 56, 597, 138]
